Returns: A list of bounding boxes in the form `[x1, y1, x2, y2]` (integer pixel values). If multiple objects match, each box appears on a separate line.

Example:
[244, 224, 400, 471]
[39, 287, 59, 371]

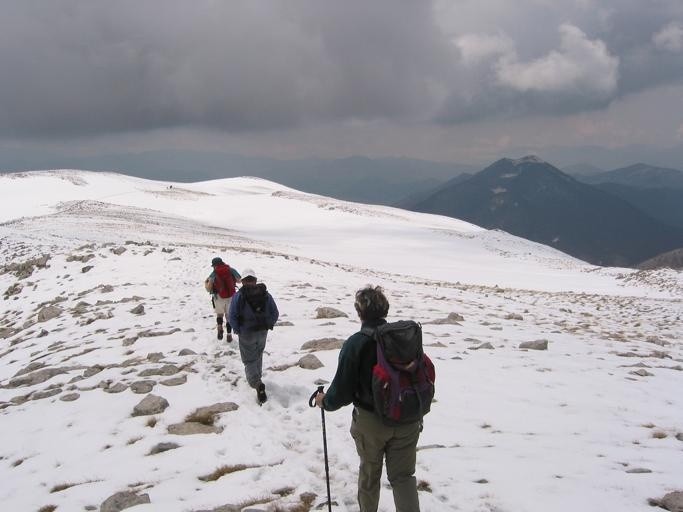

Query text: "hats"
[211, 257, 224, 267]
[241, 268, 257, 280]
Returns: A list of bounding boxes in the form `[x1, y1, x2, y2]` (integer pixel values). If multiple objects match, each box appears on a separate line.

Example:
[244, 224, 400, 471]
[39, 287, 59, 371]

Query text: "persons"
[314, 285, 424, 512]
[204, 258, 240, 343]
[228, 266, 281, 405]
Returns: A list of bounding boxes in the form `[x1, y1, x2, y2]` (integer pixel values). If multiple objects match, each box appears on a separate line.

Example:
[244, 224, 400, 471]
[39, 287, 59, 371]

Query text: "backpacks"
[358, 319, 436, 423]
[214, 264, 236, 298]
[237, 283, 273, 332]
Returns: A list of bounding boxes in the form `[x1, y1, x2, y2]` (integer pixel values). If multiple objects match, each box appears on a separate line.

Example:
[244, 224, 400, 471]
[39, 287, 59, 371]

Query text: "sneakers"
[217, 324, 223, 340]
[255, 381, 267, 403]
[227, 335, 232, 342]
